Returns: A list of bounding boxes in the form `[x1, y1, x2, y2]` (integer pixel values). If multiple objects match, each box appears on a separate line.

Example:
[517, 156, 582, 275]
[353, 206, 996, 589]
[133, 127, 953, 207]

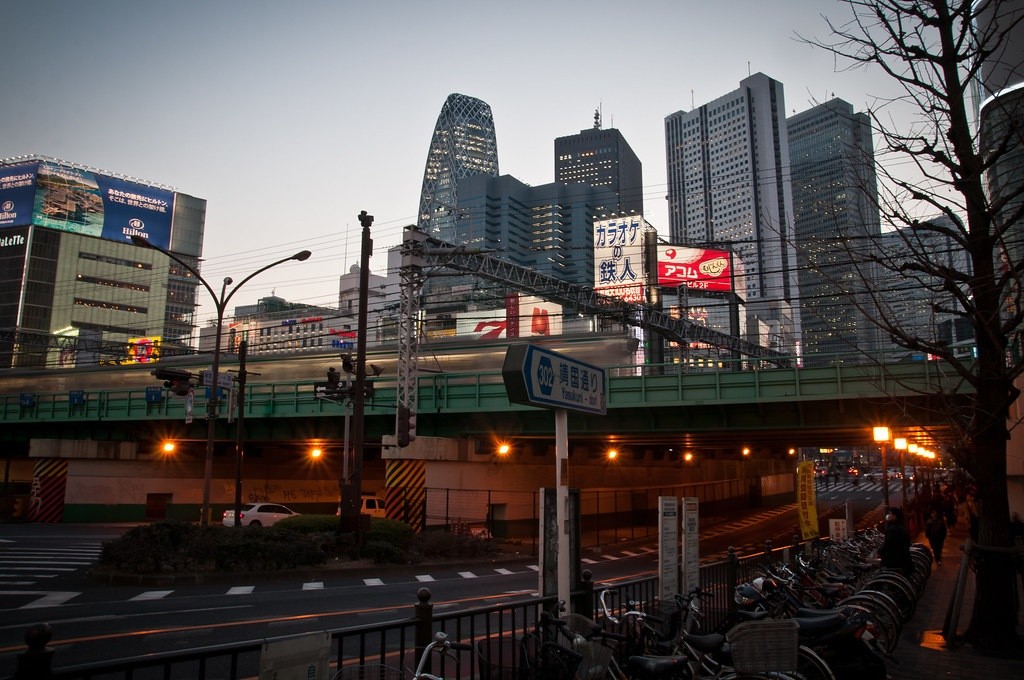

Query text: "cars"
[337, 495, 384, 518]
[223, 501, 301, 526]
[814, 458, 954, 480]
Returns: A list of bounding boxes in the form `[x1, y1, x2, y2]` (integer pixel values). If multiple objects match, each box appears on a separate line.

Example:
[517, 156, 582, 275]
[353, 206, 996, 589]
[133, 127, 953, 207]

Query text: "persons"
[815, 457, 970, 605]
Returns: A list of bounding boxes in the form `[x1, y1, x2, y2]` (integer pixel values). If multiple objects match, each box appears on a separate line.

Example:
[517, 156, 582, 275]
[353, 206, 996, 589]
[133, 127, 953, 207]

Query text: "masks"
[886, 515, 890, 520]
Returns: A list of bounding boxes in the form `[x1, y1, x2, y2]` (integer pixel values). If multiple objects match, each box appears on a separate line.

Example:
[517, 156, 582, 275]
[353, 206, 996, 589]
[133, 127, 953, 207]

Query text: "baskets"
[328, 593, 801, 680]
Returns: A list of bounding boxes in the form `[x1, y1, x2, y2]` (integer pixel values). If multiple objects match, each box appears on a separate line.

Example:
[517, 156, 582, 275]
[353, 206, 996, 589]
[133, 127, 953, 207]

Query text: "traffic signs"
[521, 345, 606, 415]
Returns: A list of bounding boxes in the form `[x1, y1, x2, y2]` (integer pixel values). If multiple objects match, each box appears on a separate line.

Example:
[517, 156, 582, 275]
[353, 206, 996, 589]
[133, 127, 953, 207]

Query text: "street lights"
[908, 443, 935, 493]
[873, 426, 893, 512]
[894, 437, 908, 510]
[131, 235, 312, 527]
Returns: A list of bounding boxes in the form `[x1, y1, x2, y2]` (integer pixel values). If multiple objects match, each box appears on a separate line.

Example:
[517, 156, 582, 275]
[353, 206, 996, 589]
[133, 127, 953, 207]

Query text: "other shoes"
[935, 559, 941, 565]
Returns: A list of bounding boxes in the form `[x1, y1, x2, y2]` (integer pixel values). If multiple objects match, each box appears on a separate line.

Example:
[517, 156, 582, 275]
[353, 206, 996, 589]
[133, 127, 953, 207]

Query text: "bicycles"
[332, 525, 934, 680]
